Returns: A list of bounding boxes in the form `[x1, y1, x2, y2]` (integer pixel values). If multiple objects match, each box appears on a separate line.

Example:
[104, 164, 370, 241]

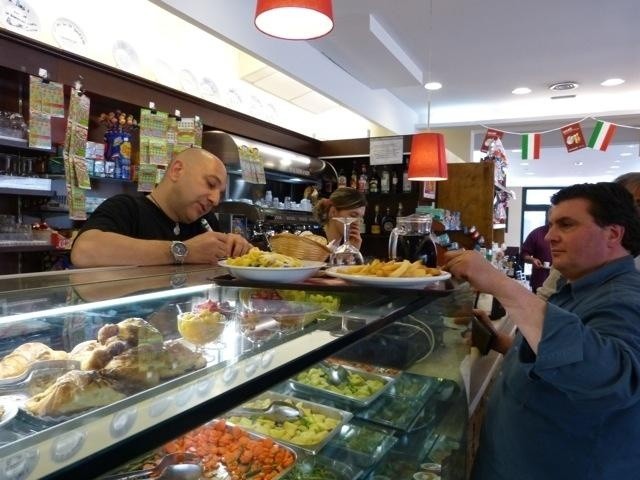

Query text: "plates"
[540, 265, 552, 269]
[325, 264, 453, 288]
[0, 398, 18, 427]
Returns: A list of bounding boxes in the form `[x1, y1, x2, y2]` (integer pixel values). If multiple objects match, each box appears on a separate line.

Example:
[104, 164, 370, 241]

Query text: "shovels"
[318, 357, 347, 385]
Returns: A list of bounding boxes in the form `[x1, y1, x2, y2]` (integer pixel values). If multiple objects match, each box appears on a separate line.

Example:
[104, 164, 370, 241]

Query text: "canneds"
[262, 189, 312, 210]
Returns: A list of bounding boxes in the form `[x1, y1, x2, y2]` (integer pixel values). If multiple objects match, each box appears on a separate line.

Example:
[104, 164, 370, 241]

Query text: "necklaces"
[150, 193, 160, 210]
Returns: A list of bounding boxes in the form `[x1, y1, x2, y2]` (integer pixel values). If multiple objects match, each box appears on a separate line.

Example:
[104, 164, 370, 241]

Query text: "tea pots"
[389, 216, 438, 269]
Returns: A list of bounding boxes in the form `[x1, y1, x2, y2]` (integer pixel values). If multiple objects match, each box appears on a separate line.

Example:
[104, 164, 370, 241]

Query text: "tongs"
[319, 358, 351, 388]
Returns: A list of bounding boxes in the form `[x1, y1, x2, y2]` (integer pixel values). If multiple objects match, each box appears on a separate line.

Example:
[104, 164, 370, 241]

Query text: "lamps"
[408, 2, 450, 183]
[252, 0, 336, 42]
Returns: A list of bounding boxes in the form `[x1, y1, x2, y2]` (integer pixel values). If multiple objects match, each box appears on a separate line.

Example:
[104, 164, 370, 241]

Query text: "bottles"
[443, 209, 463, 230]
[479, 236, 518, 279]
[84, 151, 121, 178]
[336, 158, 418, 195]
[253, 211, 322, 237]
[360, 203, 404, 236]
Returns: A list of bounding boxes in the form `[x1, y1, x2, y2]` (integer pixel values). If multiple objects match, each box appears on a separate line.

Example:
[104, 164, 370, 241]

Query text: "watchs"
[170, 240, 188, 265]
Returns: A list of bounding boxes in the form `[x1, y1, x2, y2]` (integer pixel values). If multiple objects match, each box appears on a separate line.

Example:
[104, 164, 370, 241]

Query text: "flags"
[587, 121, 616, 152]
[521, 135, 539, 160]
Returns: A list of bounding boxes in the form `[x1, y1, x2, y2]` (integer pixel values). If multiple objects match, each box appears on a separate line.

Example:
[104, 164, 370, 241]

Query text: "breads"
[0, 317, 208, 423]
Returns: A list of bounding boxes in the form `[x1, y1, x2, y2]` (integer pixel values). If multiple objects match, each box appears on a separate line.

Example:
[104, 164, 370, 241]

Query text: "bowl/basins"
[219, 258, 327, 284]
[239, 303, 325, 331]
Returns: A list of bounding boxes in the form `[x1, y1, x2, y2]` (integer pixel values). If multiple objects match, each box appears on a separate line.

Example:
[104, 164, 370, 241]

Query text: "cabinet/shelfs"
[0, 28, 433, 275]
[434, 156, 513, 254]
[0, 256, 475, 480]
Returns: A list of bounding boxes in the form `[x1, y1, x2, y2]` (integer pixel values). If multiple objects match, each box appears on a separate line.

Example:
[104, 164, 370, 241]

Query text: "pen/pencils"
[199, 217, 215, 234]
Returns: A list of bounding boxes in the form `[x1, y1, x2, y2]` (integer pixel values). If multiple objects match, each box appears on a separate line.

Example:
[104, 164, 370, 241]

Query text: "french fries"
[336, 259, 430, 277]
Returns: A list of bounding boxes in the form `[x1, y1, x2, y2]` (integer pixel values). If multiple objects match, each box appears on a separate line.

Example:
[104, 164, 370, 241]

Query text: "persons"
[69, 146, 256, 267]
[536, 171, 639, 301]
[295, 187, 362, 256]
[432, 182, 639, 479]
[520, 224, 553, 294]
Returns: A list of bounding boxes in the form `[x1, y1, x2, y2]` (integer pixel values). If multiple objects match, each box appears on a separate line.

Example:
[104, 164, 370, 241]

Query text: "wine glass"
[331, 216, 364, 265]
[176, 302, 305, 363]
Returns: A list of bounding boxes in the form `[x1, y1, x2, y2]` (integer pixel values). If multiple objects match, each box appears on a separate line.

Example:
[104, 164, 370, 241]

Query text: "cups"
[0, 153, 46, 178]
[543, 262, 550, 267]
[1, 111, 29, 139]
[259, 190, 313, 210]
[0, 213, 32, 241]
